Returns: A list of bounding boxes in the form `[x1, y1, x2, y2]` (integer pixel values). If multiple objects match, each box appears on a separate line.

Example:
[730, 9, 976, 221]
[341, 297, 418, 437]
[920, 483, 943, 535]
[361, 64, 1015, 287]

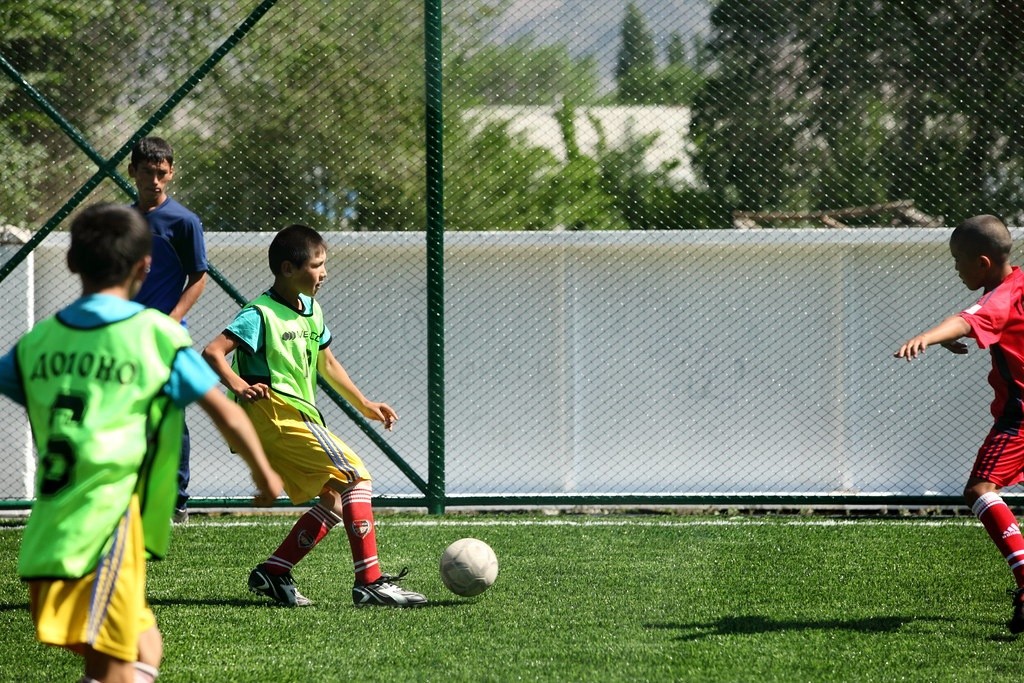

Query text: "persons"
[123, 137, 208, 525]
[0, 203, 284, 683]
[200, 226, 426, 609]
[893, 214, 1024, 634]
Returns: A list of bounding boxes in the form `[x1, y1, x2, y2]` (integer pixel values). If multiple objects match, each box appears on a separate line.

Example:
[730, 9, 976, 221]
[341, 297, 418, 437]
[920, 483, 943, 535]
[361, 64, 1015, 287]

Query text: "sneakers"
[247, 564, 318, 607]
[352, 567, 427, 609]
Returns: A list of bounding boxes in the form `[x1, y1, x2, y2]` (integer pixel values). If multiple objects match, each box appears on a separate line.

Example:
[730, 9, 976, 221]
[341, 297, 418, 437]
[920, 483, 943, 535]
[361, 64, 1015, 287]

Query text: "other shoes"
[1006, 588, 1024, 635]
[170, 508, 189, 527]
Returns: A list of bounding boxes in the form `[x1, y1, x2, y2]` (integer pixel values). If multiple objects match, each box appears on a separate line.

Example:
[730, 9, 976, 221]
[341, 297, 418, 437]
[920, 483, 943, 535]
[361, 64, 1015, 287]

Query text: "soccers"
[440, 537, 499, 596]
